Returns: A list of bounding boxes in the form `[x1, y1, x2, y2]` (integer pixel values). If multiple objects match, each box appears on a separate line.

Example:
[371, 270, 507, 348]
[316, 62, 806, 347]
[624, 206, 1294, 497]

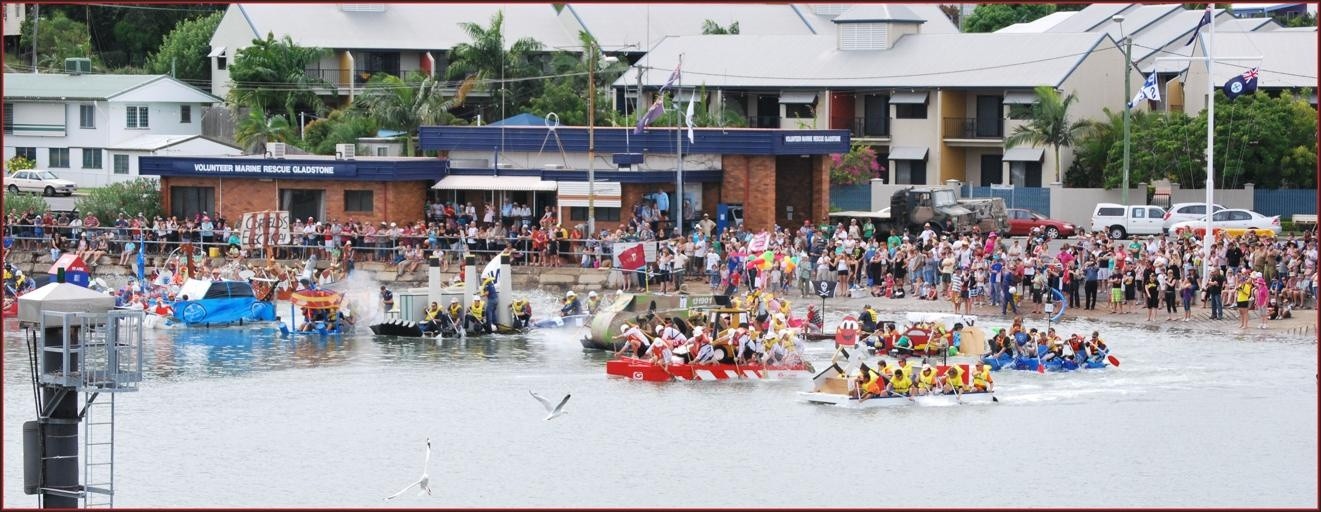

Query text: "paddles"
[949, 376, 964, 405]
[982, 374, 998, 402]
[1087, 340, 1119, 367]
[762, 344, 771, 378]
[472, 310, 492, 334]
[1035, 336, 1045, 374]
[513, 305, 521, 329]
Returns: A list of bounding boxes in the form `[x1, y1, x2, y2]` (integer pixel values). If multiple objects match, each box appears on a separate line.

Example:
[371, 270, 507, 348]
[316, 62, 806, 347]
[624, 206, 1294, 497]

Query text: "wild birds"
[525, 389, 577, 423]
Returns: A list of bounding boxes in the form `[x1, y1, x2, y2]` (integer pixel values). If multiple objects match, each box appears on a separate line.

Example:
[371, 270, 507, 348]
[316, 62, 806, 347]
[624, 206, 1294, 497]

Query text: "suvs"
[1161, 201, 1230, 231]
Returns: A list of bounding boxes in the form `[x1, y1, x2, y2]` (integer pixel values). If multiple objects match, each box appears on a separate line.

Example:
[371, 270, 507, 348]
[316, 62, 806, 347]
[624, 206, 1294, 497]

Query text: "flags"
[685, 90, 696, 145]
[1127, 71, 1161, 107]
[1182, 6, 1211, 47]
[633, 93, 665, 136]
[1223, 67, 1259, 99]
[659, 63, 681, 92]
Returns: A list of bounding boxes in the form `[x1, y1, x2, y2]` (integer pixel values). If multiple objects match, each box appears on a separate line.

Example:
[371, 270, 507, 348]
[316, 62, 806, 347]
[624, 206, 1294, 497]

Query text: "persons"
[421, 276, 499, 333]
[5, 189, 1318, 327]
[276, 307, 356, 339]
[614, 290, 821, 370]
[850, 305, 1107, 399]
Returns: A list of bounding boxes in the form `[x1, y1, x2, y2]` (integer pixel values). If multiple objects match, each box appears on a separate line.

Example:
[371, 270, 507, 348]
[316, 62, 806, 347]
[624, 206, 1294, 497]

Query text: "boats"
[4, 290, 35, 318]
[367, 317, 530, 340]
[107, 267, 359, 339]
[536, 310, 593, 330]
[980, 349, 1111, 372]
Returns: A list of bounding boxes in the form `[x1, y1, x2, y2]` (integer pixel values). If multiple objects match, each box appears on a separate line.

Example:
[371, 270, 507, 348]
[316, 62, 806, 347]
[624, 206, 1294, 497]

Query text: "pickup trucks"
[1091, 201, 1167, 240]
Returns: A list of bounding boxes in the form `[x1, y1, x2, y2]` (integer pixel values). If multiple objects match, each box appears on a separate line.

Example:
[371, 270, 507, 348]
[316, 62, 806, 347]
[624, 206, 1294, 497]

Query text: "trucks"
[879, 197, 1011, 237]
[827, 184, 976, 239]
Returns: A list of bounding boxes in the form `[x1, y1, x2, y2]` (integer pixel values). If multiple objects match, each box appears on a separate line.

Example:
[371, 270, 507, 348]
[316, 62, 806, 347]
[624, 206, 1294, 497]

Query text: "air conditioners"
[335, 144, 355, 160]
[266, 143, 285, 159]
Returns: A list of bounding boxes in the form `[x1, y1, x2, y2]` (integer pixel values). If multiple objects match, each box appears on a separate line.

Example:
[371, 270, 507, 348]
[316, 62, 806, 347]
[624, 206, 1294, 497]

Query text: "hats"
[728, 328, 737, 338]
[621, 323, 631, 334]
[976, 360, 985, 366]
[4, 209, 1317, 307]
[694, 326, 704, 337]
[778, 328, 788, 339]
[776, 313, 786, 322]
[922, 363, 932, 371]
[655, 324, 665, 336]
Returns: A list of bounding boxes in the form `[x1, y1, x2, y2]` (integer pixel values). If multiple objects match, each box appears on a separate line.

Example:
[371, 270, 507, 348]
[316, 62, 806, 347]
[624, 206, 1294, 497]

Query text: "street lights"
[580, 40, 622, 242]
[1112, 14, 1134, 203]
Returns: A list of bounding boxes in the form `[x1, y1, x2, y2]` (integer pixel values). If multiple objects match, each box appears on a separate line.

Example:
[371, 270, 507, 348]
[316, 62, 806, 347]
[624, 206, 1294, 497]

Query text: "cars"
[1168, 209, 1286, 239]
[1006, 209, 1078, 241]
[4, 168, 79, 197]
